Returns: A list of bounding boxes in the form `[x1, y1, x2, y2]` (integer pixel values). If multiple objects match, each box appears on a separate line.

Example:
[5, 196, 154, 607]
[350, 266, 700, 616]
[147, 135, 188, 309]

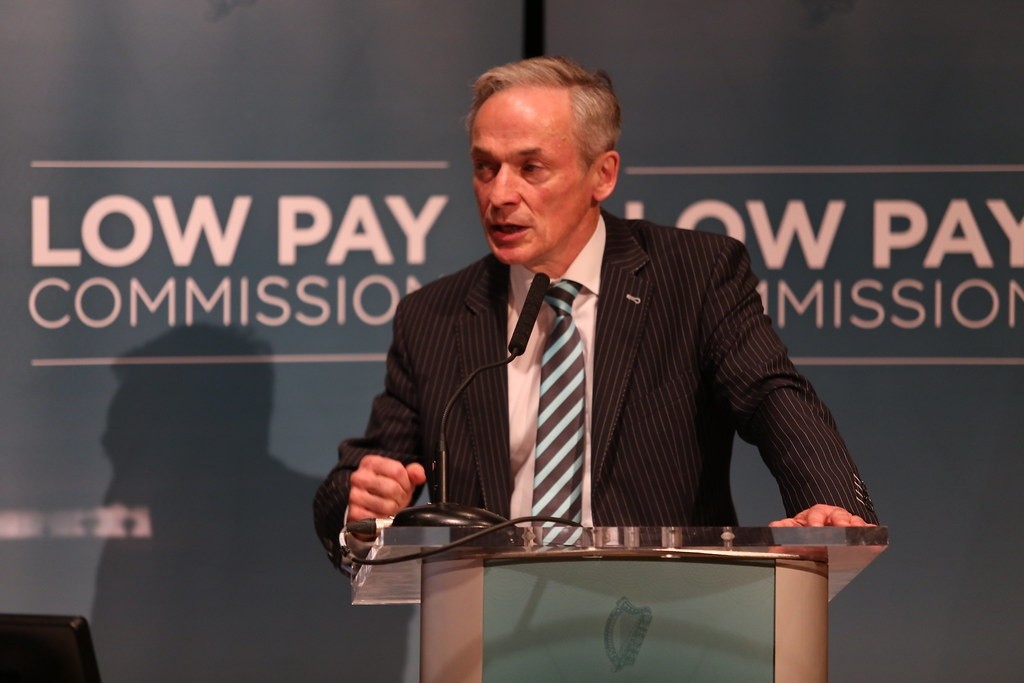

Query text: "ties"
[542, 527, 583, 548]
[530, 279, 587, 528]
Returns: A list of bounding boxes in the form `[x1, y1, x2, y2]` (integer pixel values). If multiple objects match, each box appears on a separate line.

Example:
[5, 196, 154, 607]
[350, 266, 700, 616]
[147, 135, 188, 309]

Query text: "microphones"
[392, 272, 551, 530]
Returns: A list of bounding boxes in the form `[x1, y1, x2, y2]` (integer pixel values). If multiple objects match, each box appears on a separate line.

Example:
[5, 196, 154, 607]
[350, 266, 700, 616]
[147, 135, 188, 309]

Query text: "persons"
[315, 58, 883, 571]
